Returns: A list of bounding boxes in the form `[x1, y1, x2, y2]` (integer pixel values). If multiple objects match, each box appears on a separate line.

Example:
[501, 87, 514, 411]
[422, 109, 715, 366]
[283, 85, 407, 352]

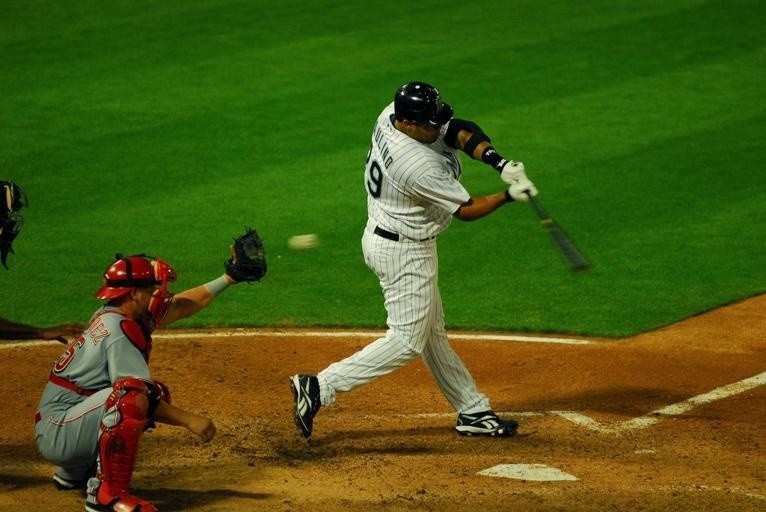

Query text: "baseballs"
[289, 234, 317, 249]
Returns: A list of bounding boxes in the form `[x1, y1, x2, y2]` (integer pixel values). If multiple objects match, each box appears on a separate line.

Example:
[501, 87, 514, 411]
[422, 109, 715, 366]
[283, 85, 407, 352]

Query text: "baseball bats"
[524, 189, 592, 270]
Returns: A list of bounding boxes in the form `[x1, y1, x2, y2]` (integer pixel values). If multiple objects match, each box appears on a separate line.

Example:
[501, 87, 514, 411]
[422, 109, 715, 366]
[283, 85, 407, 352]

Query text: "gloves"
[500, 159, 538, 201]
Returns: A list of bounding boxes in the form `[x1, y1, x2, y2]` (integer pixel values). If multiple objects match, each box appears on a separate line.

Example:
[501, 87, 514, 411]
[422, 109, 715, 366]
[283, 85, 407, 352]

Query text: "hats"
[395, 81, 454, 125]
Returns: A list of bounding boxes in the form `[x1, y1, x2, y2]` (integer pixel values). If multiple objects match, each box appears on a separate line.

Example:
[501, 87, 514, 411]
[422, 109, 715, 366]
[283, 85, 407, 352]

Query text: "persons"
[0, 181, 89, 345]
[34, 245, 237, 512]
[289, 82, 538, 438]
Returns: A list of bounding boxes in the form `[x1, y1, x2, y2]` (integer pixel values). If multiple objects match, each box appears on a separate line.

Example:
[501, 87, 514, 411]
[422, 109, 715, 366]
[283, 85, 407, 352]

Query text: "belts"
[374, 226, 435, 242]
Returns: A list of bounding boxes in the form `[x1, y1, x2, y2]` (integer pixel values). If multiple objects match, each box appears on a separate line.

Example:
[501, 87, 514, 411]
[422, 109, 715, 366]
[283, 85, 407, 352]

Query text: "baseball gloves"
[224, 227, 267, 284]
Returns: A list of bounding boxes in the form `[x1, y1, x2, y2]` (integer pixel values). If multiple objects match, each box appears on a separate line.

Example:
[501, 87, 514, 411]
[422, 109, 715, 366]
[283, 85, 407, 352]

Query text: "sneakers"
[456, 411, 519, 438]
[53, 473, 159, 512]
[288, 373, 321, 438]
[95, 253, 177, 300]
[0, 181, 28, 227]
